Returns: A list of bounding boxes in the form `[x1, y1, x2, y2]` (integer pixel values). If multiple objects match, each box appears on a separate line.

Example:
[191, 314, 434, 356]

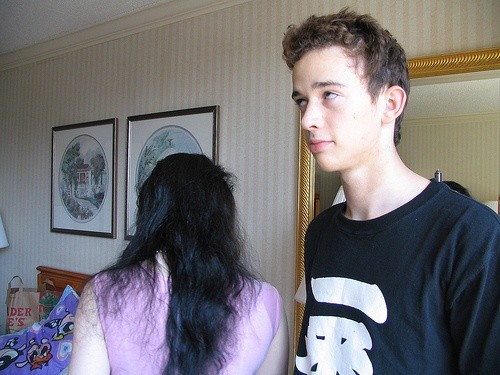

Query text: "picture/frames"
[49, 118, 117, 239]
[124, 105, 221, 241]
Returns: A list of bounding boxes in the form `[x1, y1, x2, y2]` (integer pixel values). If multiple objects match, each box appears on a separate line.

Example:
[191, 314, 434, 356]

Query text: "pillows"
[0, 285, 81, 375]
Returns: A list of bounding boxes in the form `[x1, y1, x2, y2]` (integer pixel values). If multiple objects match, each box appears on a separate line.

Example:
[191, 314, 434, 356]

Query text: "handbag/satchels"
[6, 276, 62, 334]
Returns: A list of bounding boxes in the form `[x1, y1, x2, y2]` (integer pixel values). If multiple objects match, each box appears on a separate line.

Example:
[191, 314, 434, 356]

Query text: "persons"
[283, 7, 500, 375]
[68, 152, 289, 375]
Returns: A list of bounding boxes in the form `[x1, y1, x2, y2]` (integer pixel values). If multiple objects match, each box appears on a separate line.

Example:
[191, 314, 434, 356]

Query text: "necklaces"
[156, 250, 167, 254]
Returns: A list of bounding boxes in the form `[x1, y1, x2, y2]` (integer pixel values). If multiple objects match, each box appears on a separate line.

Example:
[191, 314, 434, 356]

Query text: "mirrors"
[292, 47, 499, 375]
